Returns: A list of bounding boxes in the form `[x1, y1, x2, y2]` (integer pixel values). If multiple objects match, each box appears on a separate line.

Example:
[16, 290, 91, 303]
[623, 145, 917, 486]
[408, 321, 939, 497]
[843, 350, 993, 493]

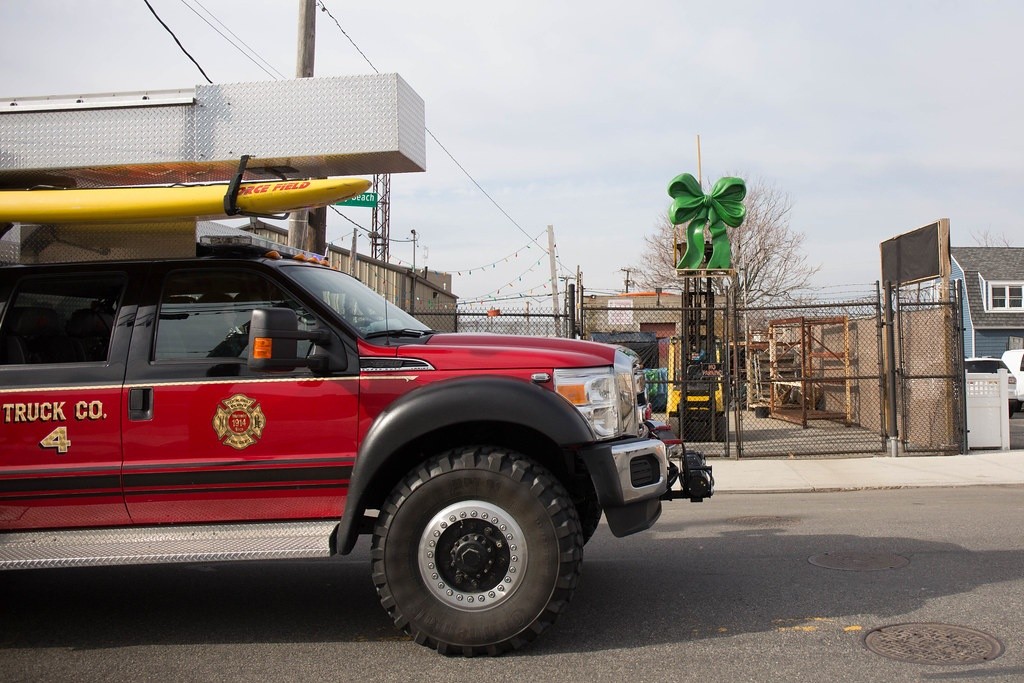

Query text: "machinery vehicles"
[666, 135, 732, 443]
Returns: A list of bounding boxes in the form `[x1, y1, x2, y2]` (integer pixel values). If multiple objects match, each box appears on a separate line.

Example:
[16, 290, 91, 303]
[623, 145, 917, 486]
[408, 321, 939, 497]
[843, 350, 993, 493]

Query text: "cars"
[1001, 349, 1024, 413]
[0, 233, 714, 657]
[911, 357, 1018, 419]
